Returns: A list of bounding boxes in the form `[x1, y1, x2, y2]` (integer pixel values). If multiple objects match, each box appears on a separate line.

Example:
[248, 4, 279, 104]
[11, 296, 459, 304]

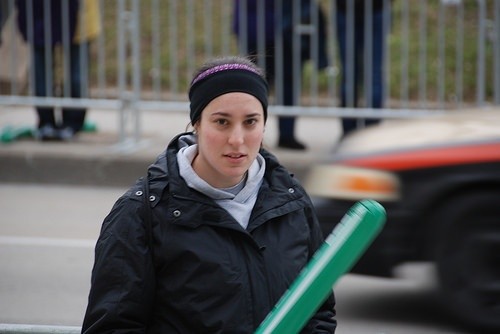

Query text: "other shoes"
[38, 124, 74, 141]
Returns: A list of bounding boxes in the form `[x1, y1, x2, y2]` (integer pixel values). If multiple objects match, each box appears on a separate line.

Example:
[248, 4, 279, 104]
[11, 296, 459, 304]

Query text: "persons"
[335, 0, 391, 139]
[13, 0, 91, 141]
[230, 0, 330, 150]
[81, 56, 337, 334]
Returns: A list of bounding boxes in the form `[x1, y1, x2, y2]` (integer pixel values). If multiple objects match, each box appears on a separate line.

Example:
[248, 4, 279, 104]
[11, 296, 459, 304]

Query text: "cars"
[300, 118, 500, 329]
